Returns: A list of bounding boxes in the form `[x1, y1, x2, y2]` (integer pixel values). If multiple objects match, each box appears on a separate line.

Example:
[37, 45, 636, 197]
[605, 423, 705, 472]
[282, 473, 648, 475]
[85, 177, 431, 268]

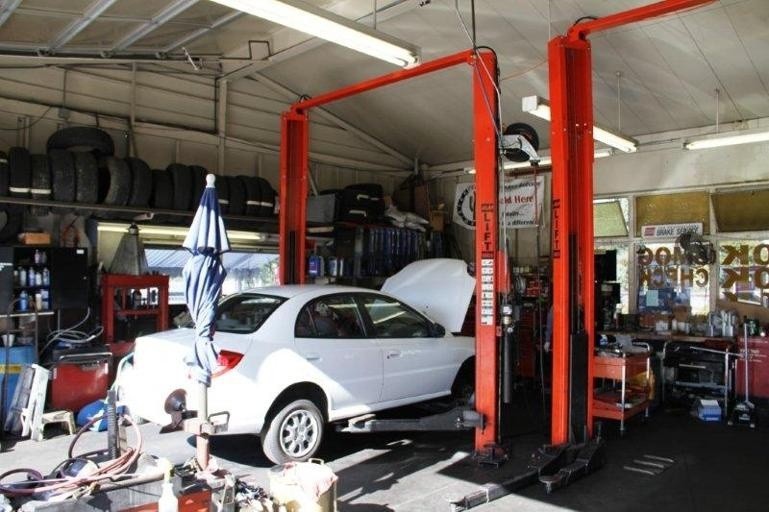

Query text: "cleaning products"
[19, 250, 51, 313]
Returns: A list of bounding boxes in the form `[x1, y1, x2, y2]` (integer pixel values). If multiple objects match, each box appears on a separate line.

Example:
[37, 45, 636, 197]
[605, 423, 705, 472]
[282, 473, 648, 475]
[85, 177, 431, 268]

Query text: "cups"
[1, 334, 15, 348]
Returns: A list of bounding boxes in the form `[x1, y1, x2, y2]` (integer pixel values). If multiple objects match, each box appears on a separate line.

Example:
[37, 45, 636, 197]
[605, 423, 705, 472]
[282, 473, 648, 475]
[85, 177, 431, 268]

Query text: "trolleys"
[659, 338, 737, 425]
[593, 337, 653, 433]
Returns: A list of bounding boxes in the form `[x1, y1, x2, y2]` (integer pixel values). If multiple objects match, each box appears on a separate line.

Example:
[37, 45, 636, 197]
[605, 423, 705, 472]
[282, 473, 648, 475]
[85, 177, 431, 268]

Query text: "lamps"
[210, 0, 423, 70]
[681, 89, 769, 150]
[467, 0, 639, 175]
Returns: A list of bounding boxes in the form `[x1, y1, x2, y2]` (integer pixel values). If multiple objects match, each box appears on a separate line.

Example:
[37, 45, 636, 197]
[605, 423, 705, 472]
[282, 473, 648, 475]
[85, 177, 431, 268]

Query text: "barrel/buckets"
[52, 364, 108, 412]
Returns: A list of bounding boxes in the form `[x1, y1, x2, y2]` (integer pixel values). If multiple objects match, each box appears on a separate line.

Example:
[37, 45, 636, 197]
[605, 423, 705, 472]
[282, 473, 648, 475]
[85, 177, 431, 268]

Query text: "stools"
[37, 409, 76, 441]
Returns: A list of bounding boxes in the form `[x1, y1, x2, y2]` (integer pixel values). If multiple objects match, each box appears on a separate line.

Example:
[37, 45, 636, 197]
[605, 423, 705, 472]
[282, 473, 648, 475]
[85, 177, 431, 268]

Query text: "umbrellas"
[181, 173, 230, 472]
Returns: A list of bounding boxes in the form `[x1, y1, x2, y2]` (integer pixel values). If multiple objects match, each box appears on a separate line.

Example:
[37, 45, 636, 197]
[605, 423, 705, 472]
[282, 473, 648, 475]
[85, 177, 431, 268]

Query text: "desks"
[597, 328, 736, 419]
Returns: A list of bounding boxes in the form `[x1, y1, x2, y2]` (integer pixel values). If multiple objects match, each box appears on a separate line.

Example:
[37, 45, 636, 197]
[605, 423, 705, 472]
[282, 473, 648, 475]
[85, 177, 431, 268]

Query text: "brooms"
[732, 322, 756, 421]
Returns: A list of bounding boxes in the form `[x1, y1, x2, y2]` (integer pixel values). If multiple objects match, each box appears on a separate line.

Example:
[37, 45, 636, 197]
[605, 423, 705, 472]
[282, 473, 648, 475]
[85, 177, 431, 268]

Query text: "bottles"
[133, 288, 141, 311]
[613, 303, 623, 329]
[13, 249, 50, 311]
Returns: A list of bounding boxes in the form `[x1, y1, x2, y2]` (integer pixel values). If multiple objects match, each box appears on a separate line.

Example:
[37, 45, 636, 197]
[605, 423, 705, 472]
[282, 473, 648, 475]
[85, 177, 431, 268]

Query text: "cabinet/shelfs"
[0, 245, 91, 319]
[45, 348, 111, 412]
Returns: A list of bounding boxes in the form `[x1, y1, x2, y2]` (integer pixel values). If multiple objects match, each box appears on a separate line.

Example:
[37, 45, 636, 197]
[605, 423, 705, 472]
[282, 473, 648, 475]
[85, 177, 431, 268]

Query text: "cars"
[128, 257, 476, 466]
